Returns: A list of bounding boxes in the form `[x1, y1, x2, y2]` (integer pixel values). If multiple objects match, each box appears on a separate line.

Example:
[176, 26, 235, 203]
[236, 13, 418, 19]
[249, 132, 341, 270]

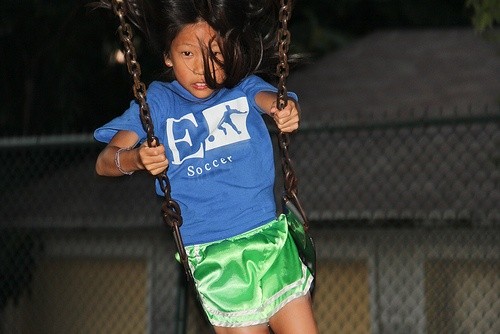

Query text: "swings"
[115, 0, 317, 327]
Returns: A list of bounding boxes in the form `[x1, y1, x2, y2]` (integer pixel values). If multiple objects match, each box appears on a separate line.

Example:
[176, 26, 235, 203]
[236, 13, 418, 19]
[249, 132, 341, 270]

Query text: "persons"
[94, 1, 320, 334]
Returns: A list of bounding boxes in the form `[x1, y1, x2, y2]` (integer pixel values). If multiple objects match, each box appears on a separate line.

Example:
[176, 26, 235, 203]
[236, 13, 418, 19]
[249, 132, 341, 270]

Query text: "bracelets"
[113, 147, 136, 175]
